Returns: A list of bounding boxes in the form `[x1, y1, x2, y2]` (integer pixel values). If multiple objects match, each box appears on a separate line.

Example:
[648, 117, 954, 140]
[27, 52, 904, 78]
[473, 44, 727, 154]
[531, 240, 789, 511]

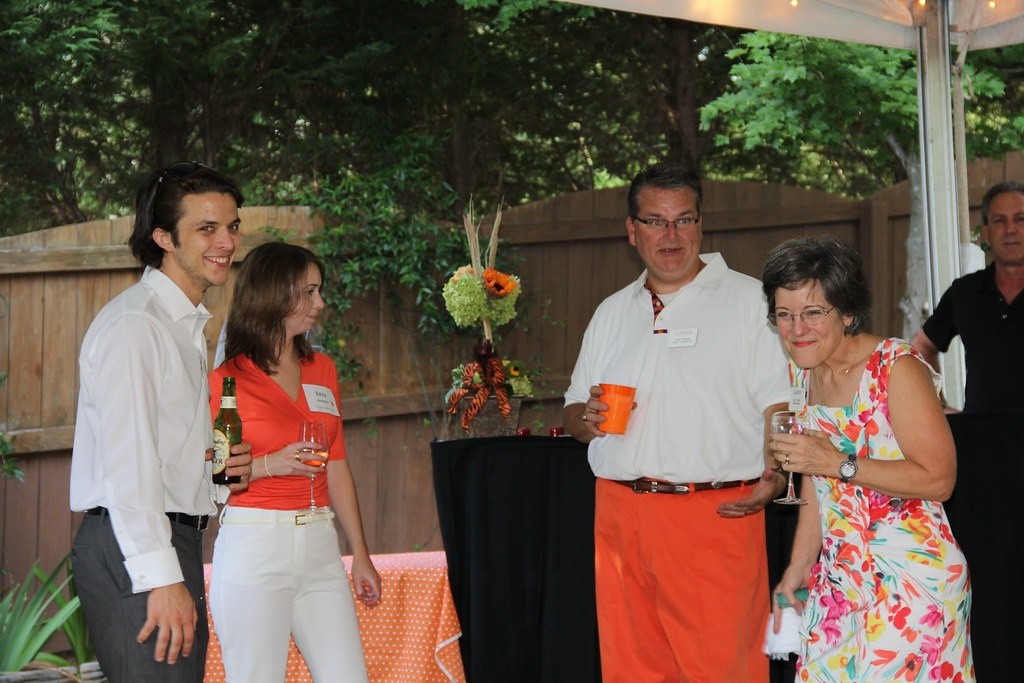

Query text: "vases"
[458, 392, 523, 437]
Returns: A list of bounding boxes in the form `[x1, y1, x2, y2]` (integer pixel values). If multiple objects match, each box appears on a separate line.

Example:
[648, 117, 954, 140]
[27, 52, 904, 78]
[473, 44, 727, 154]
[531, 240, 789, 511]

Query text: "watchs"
[839, 454, 858, 483]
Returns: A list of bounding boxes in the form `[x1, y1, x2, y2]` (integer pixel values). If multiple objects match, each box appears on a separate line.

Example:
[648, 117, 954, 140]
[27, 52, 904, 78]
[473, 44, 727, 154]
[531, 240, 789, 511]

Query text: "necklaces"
[816, 335, 858, 406]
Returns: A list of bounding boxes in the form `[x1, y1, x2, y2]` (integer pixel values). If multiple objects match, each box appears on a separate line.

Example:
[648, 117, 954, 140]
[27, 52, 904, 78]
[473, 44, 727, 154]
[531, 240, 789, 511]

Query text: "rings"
[784, 453, 790, 465]
[377, 601, 380, 604]
[582, 414, 587, 421]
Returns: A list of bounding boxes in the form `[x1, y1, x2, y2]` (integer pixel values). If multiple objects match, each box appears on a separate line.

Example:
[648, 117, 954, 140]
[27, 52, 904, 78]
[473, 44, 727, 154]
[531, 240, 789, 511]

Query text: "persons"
[763, 234, 978, 683]
[562, 162, 791, 683]
[908, 178, 1024, 683]
[209, 241, 382, 683]
[71, 162, 252, 683]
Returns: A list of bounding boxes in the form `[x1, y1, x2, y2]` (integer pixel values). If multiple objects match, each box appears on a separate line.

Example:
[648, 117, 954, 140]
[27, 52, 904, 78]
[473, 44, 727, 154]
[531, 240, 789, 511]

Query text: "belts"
[614, 478, 760, 493]
[88, 506, 209, 531]
[222, 512, 336, 524]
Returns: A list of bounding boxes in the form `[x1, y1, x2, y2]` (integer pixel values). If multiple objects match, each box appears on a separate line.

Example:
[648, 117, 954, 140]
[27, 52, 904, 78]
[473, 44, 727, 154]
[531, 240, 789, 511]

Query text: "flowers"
[440, 191, 537, 428]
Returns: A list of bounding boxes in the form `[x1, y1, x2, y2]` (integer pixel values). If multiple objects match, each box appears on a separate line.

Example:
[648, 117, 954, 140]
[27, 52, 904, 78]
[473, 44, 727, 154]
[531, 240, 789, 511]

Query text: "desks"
[203, 553, 467, 683]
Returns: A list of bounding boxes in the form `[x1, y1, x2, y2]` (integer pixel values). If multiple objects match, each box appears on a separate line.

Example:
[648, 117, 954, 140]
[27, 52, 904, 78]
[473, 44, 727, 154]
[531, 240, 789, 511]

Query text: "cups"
[596, 383, 637, 435]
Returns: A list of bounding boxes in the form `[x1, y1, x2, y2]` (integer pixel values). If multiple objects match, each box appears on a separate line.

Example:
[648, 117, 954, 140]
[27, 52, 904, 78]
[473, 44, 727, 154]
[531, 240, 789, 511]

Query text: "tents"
[569, 0, 1024, 405]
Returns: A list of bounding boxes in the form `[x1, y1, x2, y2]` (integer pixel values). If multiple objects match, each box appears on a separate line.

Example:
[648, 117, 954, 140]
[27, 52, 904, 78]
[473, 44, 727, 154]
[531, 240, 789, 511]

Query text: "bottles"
[212, 376, 242, 485]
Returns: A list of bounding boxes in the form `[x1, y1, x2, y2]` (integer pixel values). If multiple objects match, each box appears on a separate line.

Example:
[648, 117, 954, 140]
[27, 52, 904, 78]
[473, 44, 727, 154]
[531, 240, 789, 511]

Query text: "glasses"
[146, 161, 213, 227]
[635, 215, 701, 228]
[766, 307, 836, 327]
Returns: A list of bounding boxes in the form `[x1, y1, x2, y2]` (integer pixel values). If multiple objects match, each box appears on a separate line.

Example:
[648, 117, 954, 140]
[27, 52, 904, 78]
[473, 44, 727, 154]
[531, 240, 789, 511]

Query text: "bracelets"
[265, 454, 273, 478]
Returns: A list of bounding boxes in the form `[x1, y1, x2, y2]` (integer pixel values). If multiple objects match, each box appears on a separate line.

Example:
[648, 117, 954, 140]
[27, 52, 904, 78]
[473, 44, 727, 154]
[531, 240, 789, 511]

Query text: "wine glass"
[771, 411, 810, 505]
[298, 422, 330, 514]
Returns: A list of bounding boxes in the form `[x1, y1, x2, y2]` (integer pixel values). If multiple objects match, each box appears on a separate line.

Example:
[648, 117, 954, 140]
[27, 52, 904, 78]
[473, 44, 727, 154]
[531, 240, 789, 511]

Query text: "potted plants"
[0, 551, 107, 683]
